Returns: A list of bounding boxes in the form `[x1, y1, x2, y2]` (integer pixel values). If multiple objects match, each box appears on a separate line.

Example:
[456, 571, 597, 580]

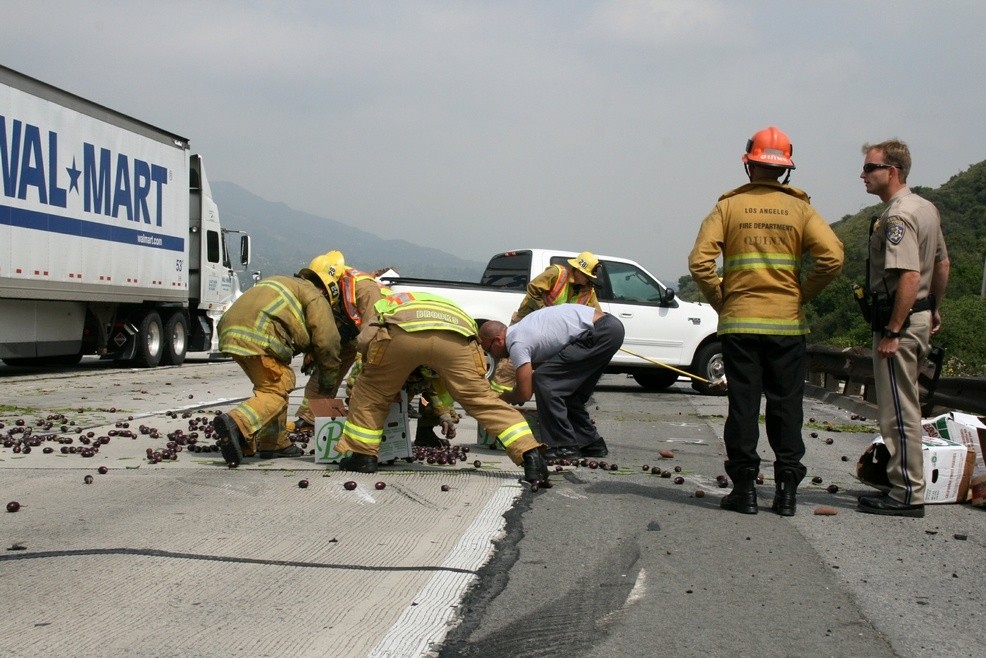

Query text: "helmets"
[567, 252, 600, 278]
[746, 126, 796, 169]
[299, 268, 339, 307]
[309, 250, 344, 282]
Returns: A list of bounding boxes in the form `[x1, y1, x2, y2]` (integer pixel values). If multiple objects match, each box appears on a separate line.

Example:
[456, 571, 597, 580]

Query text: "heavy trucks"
[0, 65, 263, 376]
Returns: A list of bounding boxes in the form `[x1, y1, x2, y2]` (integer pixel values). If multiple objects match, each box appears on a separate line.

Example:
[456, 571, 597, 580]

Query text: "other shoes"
[214, 413, 241, 464]
[414, 438, 449, 447]
[340, 452, 377, 472]
[524, 449, 548, 482]
[260, 445, 304, 458]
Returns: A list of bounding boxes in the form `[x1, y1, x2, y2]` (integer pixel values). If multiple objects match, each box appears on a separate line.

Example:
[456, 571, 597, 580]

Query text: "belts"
[910, 300, 932, 313]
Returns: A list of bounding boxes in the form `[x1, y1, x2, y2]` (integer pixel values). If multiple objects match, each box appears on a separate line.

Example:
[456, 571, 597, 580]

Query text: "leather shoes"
[858, 495, 924, 518]
[544, 445, 582, 458]
[581, 439, 607, 457]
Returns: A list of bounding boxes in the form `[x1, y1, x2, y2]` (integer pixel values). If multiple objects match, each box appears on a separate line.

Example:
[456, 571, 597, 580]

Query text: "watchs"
[882, 326, 901, 339]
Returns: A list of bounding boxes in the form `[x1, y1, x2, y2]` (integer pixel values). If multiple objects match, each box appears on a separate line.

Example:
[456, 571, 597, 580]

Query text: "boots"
[720, 459, 758, 513]
[772, 470, 796, 516]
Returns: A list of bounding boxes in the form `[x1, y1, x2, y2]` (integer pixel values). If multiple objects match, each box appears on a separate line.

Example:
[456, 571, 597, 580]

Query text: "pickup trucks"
[379, 249, 730, 398]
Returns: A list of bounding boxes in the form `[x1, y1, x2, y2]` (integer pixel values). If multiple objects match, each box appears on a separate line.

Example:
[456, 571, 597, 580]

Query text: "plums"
[0, 393, 870, 512]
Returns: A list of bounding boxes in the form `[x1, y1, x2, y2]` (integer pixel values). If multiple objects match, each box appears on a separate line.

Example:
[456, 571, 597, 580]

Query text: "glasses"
[485, 338, 495, 353]
[863, 163, 903, 172]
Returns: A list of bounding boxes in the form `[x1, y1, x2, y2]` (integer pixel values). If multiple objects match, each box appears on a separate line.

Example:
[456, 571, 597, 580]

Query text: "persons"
[479, 301, 626, 460]
[858, 137, 951, 517]
[487, 252, 602, 394]
[689, 126, 845, 517]
[213, 250, 549, 486]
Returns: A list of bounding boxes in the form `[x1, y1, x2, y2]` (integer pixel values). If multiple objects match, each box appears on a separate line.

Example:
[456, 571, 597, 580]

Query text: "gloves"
[301, 354, 313, 374]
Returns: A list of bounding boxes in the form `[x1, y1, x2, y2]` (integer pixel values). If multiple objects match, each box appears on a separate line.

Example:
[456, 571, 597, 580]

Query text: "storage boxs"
[849, 411, 986, 504]
[308, 389, 412, 463]
[477, 406, 541, 446]
